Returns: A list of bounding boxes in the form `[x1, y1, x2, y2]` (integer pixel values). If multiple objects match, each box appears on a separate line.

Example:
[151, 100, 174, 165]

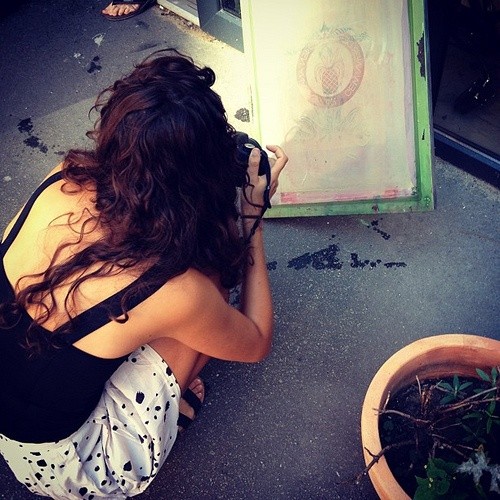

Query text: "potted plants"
[359, 333, 500, 500]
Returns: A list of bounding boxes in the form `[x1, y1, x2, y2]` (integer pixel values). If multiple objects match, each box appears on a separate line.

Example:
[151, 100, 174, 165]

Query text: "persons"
[101, 1, 157, 21]
[1, 48, 289, 500]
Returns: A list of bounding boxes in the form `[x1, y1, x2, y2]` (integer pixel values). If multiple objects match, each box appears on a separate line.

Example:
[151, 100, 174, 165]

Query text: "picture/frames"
[237, 1, 435, 219]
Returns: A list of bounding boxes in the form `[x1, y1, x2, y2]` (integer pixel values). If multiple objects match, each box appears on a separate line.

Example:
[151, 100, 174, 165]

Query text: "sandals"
[103, 0, 157, 20]
[177, 376, 203, 436]
